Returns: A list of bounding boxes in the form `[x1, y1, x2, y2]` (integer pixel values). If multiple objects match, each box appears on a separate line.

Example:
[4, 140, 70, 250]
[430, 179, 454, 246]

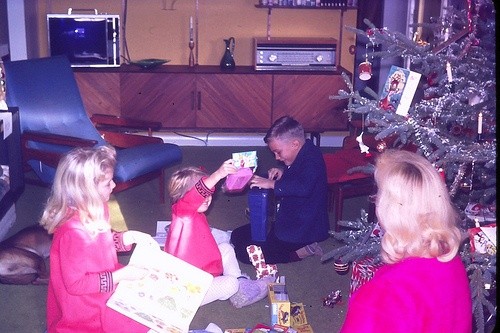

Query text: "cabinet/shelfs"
[74, 71, 352, 130]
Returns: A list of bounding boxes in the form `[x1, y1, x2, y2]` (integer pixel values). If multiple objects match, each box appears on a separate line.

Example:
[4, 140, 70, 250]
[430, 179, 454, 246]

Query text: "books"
[377, 66, 422, 116]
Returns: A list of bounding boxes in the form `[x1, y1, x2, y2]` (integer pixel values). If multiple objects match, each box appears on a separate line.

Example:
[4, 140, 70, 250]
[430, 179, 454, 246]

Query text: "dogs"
[0, 220, 54, 286]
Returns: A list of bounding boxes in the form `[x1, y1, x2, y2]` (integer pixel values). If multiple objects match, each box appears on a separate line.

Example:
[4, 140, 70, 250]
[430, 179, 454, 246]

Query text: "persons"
[164, 159, 275, 307]
[340, 150, 473, 333]
[39, 143, 222, 333]
[231, 116, 330, 264]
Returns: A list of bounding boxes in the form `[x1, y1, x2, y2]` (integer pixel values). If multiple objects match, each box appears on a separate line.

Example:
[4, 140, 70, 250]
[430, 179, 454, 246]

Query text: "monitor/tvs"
[46, 13, 119, 67]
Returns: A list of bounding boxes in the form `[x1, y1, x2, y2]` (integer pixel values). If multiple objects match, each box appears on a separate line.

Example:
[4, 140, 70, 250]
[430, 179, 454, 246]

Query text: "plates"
[132, 59, 170, 66]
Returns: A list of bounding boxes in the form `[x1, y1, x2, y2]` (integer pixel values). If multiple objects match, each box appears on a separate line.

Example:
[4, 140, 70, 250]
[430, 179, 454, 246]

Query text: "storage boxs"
[248, 169, 276, 243]
[376, 64, 422, 117]
[267, 282, 291, 327]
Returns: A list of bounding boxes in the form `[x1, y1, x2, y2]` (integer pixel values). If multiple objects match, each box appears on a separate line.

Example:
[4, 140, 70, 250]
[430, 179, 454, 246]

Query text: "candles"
[190, 16, 194, 40]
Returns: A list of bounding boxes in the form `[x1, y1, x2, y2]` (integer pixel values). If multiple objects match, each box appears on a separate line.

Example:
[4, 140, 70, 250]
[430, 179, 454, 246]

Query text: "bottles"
[219, 38, 236, 73]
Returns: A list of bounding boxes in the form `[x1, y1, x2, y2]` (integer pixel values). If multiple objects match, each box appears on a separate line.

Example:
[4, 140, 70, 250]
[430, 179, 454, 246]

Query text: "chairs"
[3, 54, 182, 202]
[324, 120, 417, 232]
